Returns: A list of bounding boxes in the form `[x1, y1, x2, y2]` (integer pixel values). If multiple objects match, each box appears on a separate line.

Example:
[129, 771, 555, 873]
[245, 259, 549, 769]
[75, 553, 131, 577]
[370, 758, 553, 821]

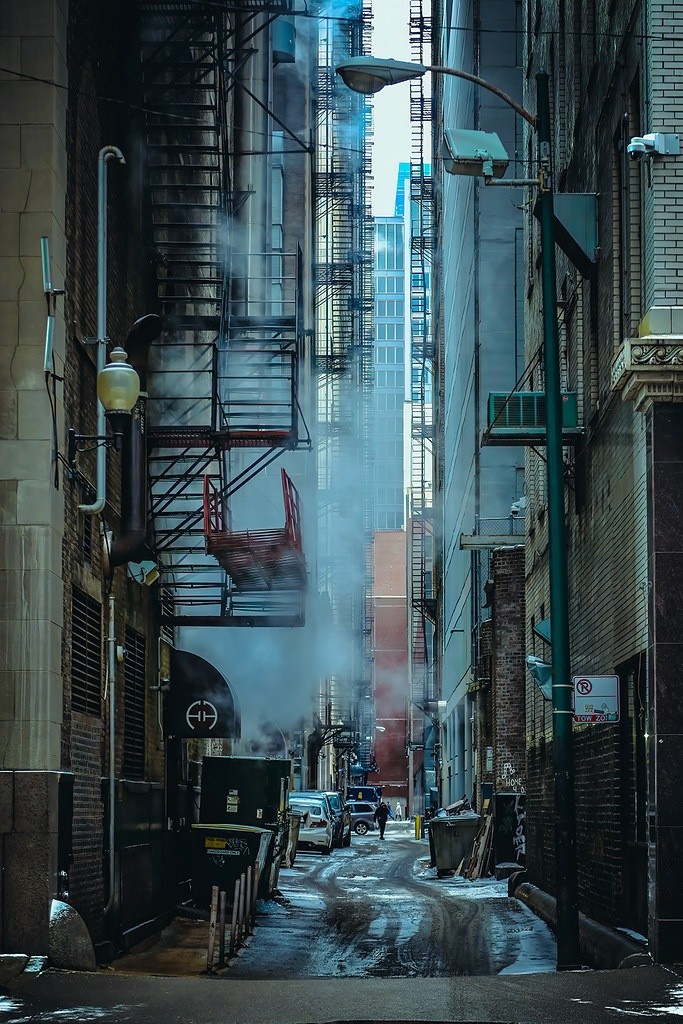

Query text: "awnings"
[164, 647, 241, 737]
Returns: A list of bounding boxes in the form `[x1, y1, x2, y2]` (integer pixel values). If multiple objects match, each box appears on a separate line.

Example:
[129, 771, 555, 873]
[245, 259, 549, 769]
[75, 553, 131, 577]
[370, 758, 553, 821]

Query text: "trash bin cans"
[426, 817, 479, 878]
[191, 823, 274, 913]
[285, 810, 305, 866]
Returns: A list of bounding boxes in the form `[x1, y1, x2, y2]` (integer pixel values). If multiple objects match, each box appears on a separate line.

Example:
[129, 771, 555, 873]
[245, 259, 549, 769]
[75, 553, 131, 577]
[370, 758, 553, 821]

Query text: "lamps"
[69, 345, 140, 472]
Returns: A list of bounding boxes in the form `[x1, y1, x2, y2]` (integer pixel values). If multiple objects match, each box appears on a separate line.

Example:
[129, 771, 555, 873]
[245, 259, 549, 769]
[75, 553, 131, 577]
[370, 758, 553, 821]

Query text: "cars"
[287, 789, 353, 856]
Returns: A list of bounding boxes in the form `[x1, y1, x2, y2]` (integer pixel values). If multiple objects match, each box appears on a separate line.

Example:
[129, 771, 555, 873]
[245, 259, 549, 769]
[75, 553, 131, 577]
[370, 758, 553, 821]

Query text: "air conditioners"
[487, 391, 546, 428]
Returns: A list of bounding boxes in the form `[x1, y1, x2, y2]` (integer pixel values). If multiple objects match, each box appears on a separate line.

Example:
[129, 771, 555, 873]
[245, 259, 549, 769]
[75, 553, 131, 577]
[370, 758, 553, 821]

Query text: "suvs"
[345, 799, 380, 836]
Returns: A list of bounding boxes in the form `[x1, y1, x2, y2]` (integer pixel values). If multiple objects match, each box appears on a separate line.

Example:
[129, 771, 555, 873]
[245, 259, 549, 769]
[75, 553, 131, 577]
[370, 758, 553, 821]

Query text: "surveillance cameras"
[510, 505, 519, 515]
[116, 645, 128, 662]
[626, 142, 646, 162]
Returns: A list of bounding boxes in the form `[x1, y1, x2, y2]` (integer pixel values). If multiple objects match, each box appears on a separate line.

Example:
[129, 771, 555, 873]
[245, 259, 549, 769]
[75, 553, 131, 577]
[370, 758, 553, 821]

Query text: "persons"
[386, 802, 394, 820]
[373, 802, 390, 840]
[394, 802, 402, 821]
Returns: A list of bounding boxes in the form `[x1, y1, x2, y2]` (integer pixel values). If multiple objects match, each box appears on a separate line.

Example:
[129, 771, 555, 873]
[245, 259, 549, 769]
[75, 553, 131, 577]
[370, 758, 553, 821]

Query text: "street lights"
[332, 54, 583, 973]
